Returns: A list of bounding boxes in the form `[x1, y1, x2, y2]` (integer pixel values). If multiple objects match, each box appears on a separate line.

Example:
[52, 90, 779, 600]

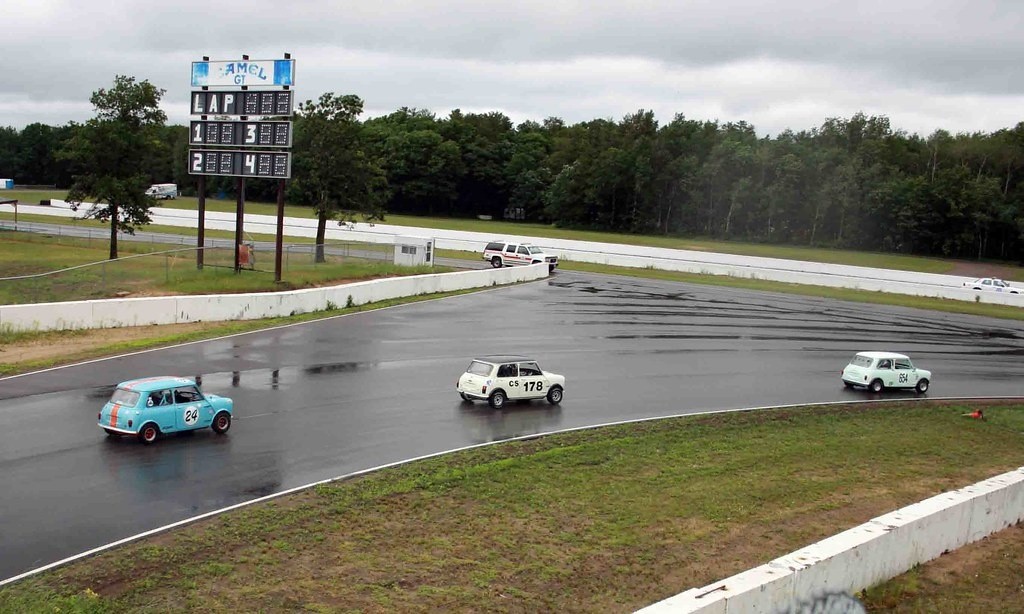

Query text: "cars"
[455, 354, 567, 408]
[145, 182, 178, 199]
[840, 350, 933, 396]
[97, 374, 235, 445]
[482, 240, 559, 272]
[963, 275, 1024, 295]
